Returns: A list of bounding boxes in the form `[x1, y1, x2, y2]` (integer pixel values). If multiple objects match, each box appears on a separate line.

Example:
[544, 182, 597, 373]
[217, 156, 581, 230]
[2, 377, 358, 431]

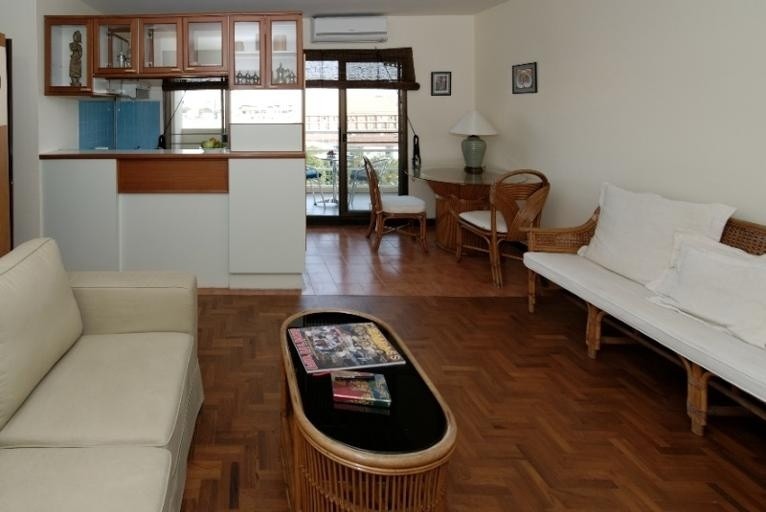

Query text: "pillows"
[577, 179, 738, 293]
[646, 232, 766, 350]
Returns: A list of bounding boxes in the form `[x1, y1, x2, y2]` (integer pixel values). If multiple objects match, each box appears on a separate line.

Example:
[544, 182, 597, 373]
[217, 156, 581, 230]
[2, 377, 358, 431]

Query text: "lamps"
[447, 108, 497, 173]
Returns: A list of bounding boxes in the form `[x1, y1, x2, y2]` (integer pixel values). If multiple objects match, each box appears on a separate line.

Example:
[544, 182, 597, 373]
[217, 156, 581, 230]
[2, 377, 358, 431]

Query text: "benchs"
[516, 179, 766, 436]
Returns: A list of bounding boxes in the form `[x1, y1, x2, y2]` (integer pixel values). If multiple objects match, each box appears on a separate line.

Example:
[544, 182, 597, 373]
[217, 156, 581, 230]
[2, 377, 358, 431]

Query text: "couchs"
[0, 236, 204, 511]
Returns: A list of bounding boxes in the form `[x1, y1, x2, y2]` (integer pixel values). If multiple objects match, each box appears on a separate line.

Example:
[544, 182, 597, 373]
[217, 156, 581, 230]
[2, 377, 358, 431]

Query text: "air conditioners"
[310, 15, 388, 43]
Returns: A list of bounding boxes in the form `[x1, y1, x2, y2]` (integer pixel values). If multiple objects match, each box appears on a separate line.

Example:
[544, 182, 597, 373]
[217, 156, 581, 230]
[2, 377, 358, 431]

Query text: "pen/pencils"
[335, 376, 375, 381]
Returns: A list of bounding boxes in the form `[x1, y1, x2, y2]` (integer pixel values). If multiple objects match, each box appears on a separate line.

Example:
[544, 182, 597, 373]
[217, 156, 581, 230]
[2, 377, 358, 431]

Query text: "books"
[334, 403, 390, 417]
[287, 321, 406, 374]
[330, 369, 391, 408]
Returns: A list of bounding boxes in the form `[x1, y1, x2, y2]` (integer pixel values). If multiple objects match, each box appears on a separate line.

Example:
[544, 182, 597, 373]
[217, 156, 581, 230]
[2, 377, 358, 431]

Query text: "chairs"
[457, 169, 550, 291]
[349, 158, 387, 204]
[305, 166, 327, 207]
[362, 155, 429, 254]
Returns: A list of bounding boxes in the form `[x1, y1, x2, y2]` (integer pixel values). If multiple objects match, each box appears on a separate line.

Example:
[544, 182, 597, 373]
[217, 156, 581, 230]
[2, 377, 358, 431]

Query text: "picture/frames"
[511, 62, 537, 93]
[431, 71, 452, 96]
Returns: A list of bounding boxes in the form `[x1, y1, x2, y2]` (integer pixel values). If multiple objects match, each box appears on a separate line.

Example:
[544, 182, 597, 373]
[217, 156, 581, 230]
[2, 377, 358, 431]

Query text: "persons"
[68, 30, 83, 83]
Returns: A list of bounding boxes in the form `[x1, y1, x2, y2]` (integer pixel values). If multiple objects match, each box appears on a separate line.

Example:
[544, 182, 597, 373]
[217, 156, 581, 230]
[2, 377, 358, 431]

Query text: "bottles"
[119, 50, 125, 68]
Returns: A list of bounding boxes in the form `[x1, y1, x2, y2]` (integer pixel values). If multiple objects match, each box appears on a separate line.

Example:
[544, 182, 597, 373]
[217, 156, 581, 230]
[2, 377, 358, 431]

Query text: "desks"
[314, 153, 352, 204]
[405, 165, 529, 255]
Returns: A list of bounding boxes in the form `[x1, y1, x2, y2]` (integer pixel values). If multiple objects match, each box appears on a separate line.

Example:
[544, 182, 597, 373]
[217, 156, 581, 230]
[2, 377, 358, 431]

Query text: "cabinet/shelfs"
[44, 9, 303, 98]
